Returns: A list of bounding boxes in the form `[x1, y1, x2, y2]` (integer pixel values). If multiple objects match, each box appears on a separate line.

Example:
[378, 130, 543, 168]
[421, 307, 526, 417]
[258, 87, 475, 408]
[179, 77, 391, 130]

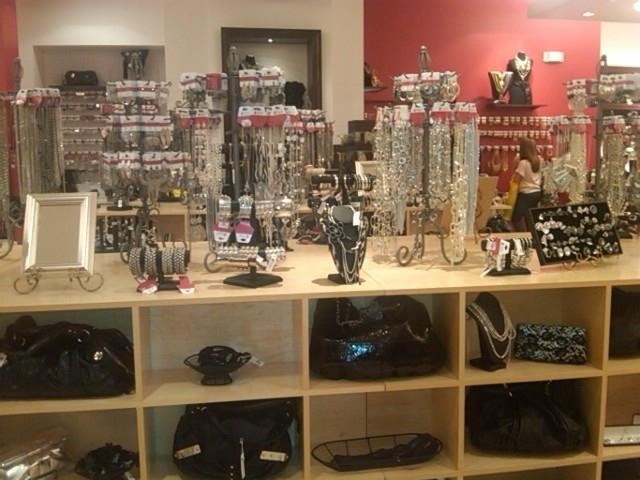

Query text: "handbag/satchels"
[469, 387, 587, 453]
[3, 321, 134, 400]
[312, 297, 445, 382]
[172, 400, 299, 480]
[2, 425, 71, 479]
[514, 321, 589, 365]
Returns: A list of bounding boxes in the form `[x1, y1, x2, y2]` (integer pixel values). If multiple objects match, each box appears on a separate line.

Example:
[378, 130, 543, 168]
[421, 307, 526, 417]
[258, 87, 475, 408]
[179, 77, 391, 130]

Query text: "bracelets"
[163, 247, 172, 275]
[144, 245, 158, 276]
[172, 248, 187, 274]
[129, 247, 143, 277]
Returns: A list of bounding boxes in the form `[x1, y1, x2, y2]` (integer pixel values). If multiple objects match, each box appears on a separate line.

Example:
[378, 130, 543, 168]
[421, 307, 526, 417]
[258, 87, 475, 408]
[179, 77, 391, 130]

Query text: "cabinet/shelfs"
[0, 232, 640, 480]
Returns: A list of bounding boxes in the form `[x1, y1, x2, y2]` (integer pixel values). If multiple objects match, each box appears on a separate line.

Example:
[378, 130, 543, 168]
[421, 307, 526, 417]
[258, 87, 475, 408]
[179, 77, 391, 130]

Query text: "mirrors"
[219, 26, 323, 188]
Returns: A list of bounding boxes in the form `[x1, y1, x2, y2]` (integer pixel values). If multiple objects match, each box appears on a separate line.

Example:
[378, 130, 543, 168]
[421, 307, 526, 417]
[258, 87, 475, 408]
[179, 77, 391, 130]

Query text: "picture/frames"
[20, 191, 97, 279]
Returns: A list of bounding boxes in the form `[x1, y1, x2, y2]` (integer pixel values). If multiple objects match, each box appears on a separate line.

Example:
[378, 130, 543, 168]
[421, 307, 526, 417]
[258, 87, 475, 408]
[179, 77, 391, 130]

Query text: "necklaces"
[371, 99, 479, 265]
[240, 120, 334, 202]
[602, 132, 640, 218]
[490, 70, 511, 101]
[320, 205, 367, 285]
[515, 57, 530, 80]
[491, 150, 509, 172]
[0, 95, 66, 220]
[568, 132, 588, 203]
[469, 301, 517, 375]
[241, 62, 267, 69]
